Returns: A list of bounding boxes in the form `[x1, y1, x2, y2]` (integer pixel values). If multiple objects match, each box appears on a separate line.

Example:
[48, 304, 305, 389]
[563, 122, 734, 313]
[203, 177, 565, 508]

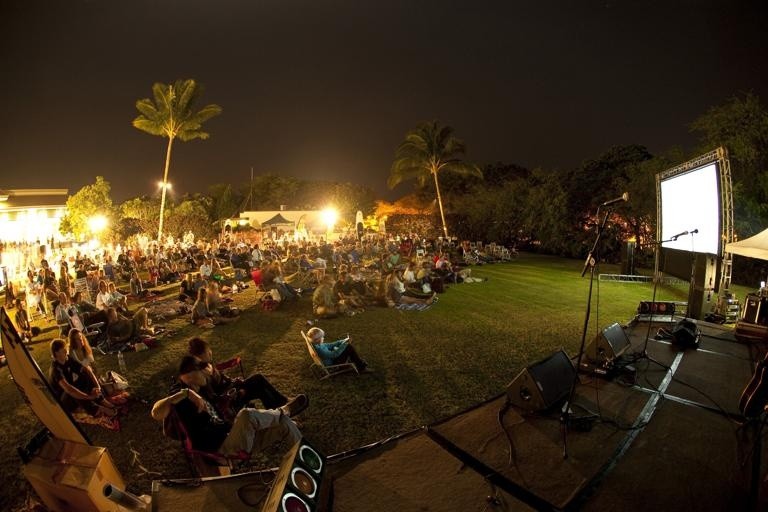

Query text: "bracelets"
[180, 387, 189, 399]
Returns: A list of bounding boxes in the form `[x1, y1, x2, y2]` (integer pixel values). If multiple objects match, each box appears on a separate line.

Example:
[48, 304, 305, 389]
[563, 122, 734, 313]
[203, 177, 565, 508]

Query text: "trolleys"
[719, 296, 740, 323]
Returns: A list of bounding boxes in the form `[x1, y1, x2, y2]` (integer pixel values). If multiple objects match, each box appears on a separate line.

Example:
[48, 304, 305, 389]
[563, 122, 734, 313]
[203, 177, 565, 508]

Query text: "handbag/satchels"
[98, 371, 129, 394]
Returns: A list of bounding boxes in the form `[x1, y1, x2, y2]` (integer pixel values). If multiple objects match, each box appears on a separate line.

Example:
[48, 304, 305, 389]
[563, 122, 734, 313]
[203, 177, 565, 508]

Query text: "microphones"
[604, 192, 630, 205]
[690, 230, 698, 234]
[672, 230, 688, 237]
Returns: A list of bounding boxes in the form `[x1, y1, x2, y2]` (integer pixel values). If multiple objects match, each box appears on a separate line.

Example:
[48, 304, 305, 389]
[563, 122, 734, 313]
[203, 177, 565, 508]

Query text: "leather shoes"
[287, 394, 309, 418]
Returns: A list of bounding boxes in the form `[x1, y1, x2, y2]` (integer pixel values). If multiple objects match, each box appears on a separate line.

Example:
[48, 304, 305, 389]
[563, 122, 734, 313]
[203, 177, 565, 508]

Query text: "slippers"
[291, 418, 306, 431]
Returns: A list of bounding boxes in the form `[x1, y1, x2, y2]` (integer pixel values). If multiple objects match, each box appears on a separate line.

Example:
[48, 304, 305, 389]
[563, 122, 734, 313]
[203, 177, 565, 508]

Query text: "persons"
[66, 328, 95, 374]
[186, 336, 309, 431]
[151, 354, 309, 467]
[304, 327, 376, 373]
[46, 338, 123, 417]
[0, 225, 520, 354]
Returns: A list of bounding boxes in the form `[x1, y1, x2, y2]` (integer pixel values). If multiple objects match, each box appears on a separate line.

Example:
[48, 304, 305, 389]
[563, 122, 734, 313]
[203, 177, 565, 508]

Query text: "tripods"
[539, 211, 608, 458]
[619, 236, 672, 372]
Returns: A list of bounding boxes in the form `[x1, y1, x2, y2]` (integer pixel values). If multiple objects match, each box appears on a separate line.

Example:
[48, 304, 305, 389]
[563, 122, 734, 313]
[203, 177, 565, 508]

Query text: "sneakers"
[365, 363, 378, 371]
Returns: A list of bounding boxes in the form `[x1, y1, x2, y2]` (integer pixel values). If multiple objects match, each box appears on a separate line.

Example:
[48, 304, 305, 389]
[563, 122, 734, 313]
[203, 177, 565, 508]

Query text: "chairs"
[170, 356, 251, 477]
[299, 330, 359, 382]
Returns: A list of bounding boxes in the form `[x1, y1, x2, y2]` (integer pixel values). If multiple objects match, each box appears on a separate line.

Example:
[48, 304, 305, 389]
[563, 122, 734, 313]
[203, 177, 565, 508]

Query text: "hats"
[306, 327, 325, 342]
[177, 354, 208, 375]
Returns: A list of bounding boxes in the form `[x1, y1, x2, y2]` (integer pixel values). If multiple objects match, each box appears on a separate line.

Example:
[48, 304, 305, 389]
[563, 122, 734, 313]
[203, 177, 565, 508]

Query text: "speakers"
[584, 322, 630, 366]
[671, 319, 701, 351]
[507, 348, 580, 414]
